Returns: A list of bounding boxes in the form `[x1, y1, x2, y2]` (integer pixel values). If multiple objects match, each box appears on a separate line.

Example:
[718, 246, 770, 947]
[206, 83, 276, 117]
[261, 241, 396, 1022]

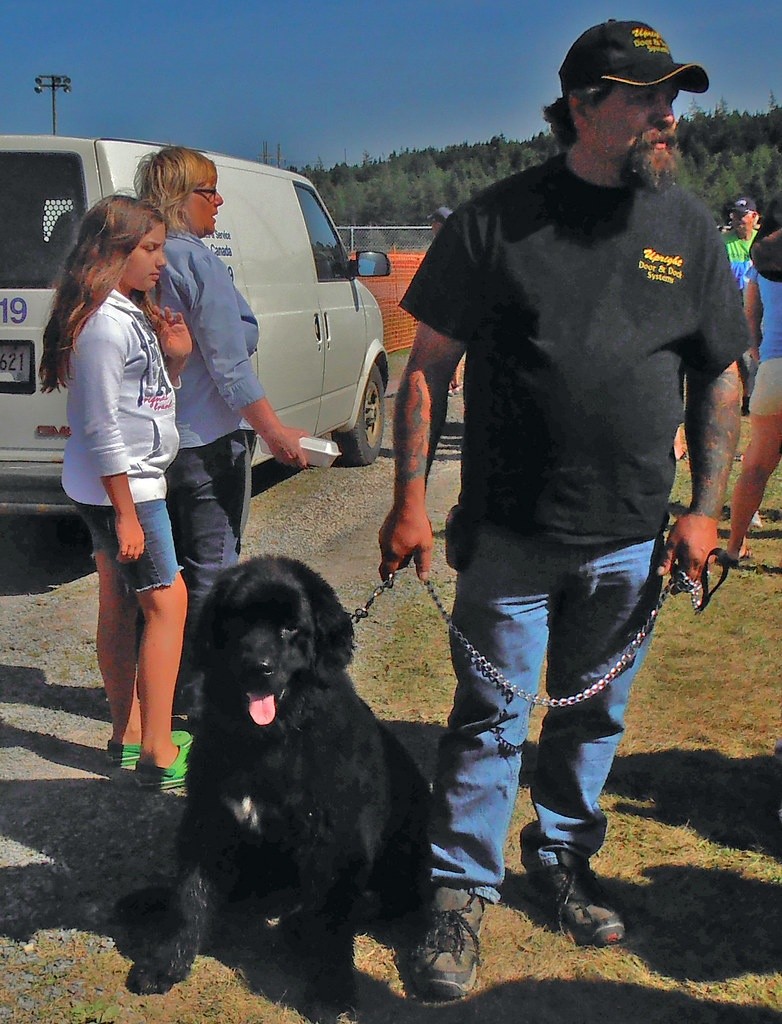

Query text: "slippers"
[725, 545, 754, 562]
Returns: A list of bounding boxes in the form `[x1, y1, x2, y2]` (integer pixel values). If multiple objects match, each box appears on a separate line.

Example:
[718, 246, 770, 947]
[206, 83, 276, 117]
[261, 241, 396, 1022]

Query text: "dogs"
[126, 555, 435, 1024]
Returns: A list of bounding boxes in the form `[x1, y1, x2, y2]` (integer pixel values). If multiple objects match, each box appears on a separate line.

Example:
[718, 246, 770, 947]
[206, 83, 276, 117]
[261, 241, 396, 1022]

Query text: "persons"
[374, 18, 782, 999]
[41, 145, 312, 793]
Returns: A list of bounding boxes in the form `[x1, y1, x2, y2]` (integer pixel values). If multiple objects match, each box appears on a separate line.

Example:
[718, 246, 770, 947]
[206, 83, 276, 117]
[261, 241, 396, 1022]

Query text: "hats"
[559, 18, 710, 93]
[427, 207, 454, 224]
[731, 197, 756, 213]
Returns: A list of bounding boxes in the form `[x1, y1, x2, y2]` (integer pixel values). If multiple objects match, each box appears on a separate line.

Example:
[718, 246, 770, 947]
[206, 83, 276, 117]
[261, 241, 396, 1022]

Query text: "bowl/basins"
[257, 430, 343, 471]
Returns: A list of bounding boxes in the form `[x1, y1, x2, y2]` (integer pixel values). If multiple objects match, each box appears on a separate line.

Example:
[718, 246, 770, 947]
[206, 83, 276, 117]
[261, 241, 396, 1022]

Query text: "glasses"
[192, 188, 217, 202]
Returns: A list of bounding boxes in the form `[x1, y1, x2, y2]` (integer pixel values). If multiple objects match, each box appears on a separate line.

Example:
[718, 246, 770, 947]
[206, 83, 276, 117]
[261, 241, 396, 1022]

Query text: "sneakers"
[411, 885, 486, 994]
[527, 863, 626, 947]
[107, 729, 195, 766]
[135, 745, 190, 790]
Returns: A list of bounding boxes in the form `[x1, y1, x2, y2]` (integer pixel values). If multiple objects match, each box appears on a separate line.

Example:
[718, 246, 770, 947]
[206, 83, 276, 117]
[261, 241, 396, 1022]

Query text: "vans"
[0, 128, 394, 518]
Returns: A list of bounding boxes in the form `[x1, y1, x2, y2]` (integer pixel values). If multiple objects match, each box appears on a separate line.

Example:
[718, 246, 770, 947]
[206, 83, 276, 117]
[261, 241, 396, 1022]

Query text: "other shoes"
[750, 511, 762, 528]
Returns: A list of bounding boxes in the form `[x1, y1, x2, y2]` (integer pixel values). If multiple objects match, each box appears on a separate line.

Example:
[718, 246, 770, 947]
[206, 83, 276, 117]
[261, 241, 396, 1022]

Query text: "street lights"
[32, 72, 76, 136]
